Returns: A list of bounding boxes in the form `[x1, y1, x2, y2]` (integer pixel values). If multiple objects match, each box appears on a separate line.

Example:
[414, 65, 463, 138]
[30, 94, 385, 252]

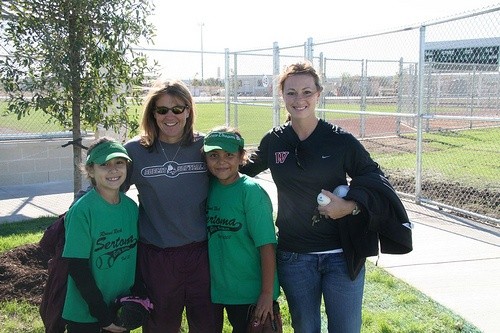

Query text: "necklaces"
[158, 137, 183, 171]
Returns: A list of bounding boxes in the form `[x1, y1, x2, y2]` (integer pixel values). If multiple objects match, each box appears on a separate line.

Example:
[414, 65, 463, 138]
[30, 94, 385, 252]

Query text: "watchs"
[352, 205, 360, 215]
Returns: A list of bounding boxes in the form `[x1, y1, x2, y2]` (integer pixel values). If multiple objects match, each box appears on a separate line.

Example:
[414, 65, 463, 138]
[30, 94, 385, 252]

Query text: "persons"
[61, 136, 139, 333]
[119, 79, 213, 333]
[239, 59, 386, 333]
[201, 125, 282, 333]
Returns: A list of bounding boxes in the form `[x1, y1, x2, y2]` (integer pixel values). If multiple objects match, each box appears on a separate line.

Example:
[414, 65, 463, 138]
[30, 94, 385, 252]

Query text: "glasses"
[154, 104, 188, 115]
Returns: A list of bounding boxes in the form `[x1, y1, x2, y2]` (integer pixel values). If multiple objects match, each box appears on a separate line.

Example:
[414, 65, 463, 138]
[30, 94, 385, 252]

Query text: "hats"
[86, 141, 132, 164]
[203, 132, 244, 154]
